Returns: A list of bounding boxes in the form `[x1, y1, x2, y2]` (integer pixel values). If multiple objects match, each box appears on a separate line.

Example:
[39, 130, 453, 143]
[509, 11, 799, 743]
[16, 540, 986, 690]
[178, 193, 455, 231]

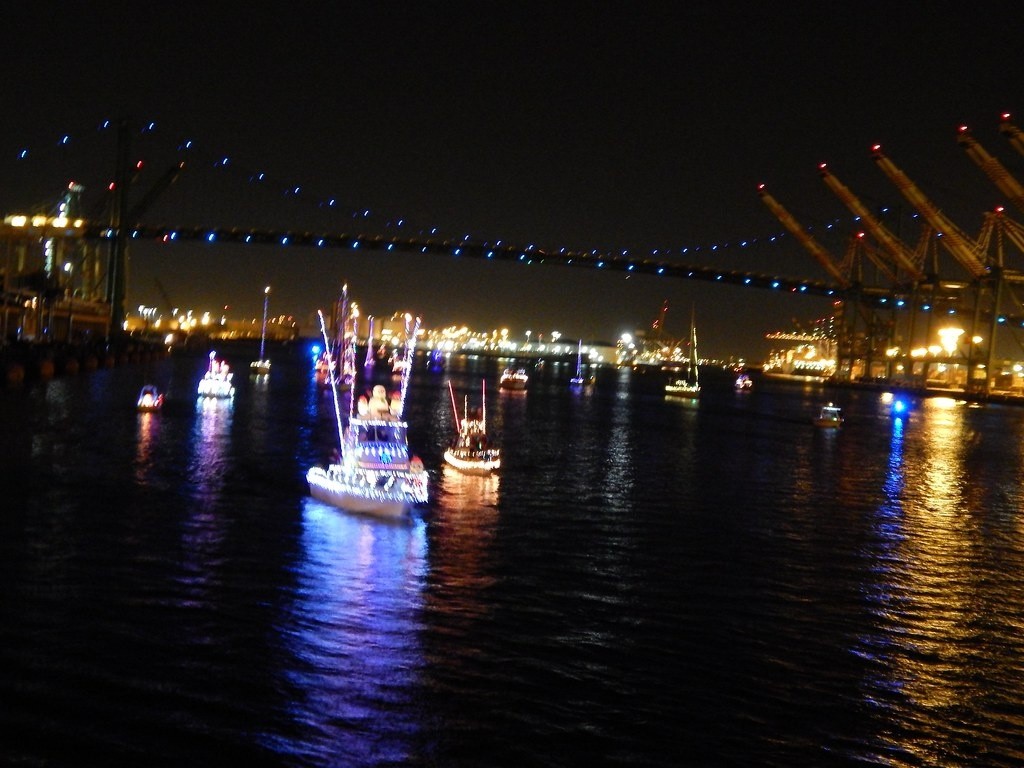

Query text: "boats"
[376, 344, 387, 361]
[499, 366, 530, 389]
[444, 379, 504, 476]
[364, 316, 377, 368]
[813, 405, 845, 427]
[388, 348, 404, 374]
[734, 373, 754, 391]
[196, 350, 235, 399]
[428, 349, 445, 373]
[303, 306, 431, 519]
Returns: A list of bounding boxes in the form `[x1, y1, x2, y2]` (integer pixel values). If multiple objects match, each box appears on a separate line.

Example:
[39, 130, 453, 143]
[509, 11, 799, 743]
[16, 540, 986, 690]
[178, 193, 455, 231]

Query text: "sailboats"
[314, 284, 358, 392]
[250, 292, 272, 375]
[571, 340, 597, 387]
[664, 299, 703, 399]
